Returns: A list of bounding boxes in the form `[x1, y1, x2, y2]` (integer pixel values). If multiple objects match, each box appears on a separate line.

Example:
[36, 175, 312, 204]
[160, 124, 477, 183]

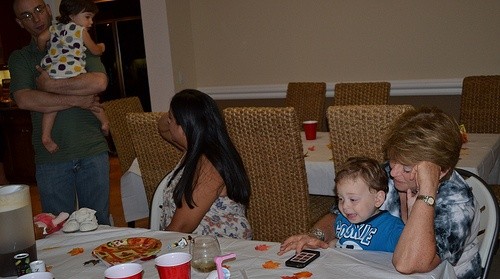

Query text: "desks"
[118, 130, 500, 229]
[0, 218, 456, 279]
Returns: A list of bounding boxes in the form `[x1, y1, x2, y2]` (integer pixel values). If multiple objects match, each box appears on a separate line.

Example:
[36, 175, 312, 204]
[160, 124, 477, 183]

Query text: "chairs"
[455, 167, 496, 279]
[459, 75, 500, 133]
[98, 72, 415, 242]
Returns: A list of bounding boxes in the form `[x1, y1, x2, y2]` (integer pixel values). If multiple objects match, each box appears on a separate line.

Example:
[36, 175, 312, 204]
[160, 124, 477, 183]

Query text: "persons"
[41, 0, 109, 154]
[9, 0, 111, 226]
[277, 107, 482, 279]
[158, 89, 253, 240]
[328, 156, 405, 253]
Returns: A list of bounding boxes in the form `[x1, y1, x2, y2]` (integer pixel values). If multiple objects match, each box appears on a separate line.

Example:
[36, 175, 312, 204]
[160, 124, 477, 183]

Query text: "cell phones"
[286, 249, 320, 268]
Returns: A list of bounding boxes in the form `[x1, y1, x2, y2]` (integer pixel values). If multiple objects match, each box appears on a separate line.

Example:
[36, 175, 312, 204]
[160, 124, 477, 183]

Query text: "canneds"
[14, 253, 32, 277]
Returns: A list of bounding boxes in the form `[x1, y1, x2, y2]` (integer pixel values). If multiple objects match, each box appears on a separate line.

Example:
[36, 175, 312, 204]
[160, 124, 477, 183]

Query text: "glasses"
[16, 4, 47, 21]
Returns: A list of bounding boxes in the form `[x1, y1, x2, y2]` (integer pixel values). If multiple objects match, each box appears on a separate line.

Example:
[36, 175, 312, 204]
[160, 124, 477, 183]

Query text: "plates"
[191, 260, 230, 279]
[91, 237, 162, 265]
[17, 272, 54, 279]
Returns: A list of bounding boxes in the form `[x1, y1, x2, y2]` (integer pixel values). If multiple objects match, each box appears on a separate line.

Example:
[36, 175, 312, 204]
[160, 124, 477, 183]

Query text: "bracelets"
[308, 228, 325, 241]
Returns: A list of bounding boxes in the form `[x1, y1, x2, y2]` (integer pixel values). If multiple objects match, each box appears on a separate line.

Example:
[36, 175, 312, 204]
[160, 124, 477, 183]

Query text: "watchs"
[417, 195, 436, 209]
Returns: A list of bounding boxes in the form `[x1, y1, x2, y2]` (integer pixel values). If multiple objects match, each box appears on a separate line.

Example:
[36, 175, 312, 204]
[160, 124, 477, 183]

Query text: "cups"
[189, 235, 221, 273]
[0, 184, 38, 277]
[302, 120, 318, 140]
[103, 263, 144, 279]
[29, 259, 46, 272]
[153, 252, 193, 279]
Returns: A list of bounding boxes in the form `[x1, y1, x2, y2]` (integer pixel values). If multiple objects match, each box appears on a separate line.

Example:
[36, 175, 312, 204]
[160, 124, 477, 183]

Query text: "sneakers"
[62, 211, 79, 232]
[80, 208, 97, 232]
